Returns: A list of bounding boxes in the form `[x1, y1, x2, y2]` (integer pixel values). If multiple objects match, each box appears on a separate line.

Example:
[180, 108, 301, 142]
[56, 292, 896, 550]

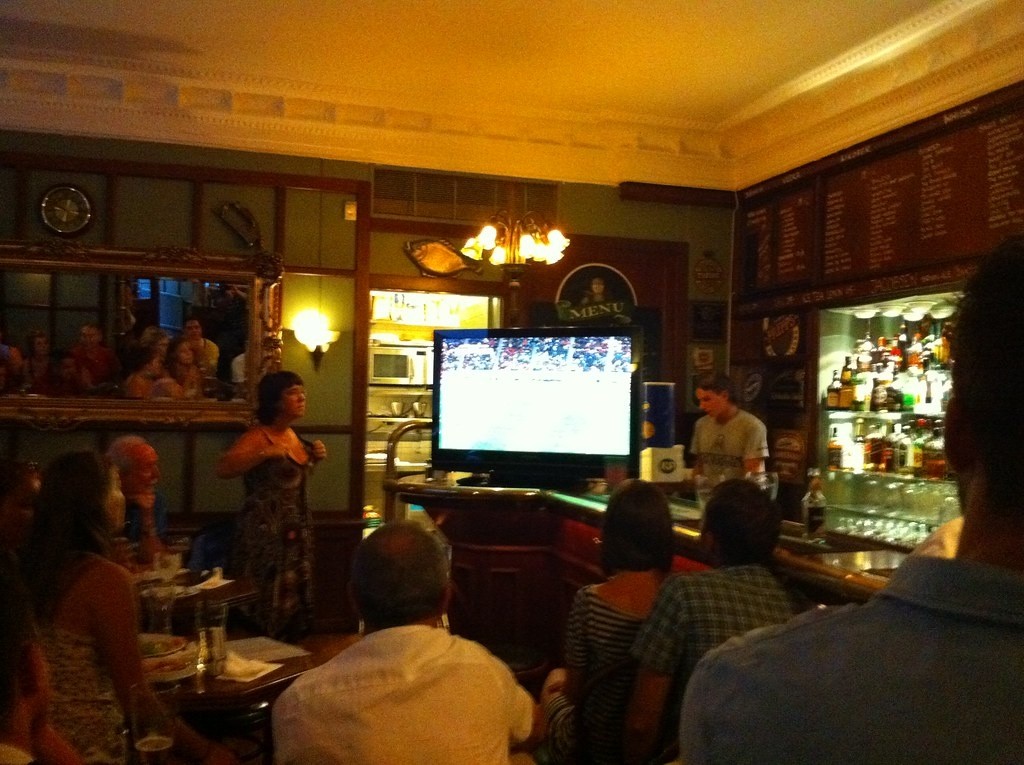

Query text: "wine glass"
[155, 553, 181, 586]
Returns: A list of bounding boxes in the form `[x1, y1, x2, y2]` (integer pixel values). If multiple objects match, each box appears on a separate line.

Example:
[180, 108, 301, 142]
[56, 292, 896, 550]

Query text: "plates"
[136, 632, 196, 681]
[141, 585, 202, 600]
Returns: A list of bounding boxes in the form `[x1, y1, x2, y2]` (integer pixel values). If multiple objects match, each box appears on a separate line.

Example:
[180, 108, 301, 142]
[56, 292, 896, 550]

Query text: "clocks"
[39, 182, 96, 236]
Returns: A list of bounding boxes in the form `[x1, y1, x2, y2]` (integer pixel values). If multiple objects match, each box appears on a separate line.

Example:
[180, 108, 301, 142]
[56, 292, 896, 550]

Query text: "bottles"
[828, 317, 957, 479]
[801, 468, 828, 546]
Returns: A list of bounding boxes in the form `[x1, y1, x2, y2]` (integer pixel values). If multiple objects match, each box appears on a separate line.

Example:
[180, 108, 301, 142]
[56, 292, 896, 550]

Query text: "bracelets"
[258, 450, 268, 458]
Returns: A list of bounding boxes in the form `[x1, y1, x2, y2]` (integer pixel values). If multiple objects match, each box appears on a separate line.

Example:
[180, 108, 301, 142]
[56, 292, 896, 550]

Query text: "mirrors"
[0, 239, 286, 434]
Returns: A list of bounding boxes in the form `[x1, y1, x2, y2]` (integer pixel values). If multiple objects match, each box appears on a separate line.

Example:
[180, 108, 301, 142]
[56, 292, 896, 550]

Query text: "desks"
[143, 643, 319, 764]
[130, 564, 265, 612]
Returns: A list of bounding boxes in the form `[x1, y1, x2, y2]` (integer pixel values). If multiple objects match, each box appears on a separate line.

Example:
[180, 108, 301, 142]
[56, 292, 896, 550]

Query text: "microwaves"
[368, 343, 437, 386]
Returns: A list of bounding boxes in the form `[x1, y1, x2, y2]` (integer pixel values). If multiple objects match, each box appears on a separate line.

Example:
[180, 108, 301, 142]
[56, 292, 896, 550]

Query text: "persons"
[624, 480, 797, 765]
[270, 520, 544, 765]
[679, 234, 1024, 765]
[218, 371, 326, 634]
[688, 371, 769, 507]
[543, 480, 675, 765]
[0, 435, 242, 765]
[0, 317, 249, 400]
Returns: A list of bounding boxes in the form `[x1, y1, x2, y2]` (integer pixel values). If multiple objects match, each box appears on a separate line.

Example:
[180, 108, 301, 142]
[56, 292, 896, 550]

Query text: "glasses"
[286, 389, 308, 401]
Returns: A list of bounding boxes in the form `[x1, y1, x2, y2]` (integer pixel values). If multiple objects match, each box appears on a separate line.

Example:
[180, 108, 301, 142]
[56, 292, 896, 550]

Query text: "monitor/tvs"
[432, 326, 639, 490]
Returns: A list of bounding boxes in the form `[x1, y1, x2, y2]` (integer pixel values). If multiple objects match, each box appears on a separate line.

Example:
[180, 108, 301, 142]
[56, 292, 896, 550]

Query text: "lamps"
[460, 214, 573, 291]
[295, 330, 341, 369]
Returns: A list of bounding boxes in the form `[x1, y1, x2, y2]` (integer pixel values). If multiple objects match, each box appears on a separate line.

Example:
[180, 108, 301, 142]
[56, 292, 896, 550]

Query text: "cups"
[130, 682, 181, 765]
[147, 582, 175, 635]
[694, 475, 727, 528]
[743, 472, 779, 500]
[193, 601, 226, 676]
[412, 399, 427, 416]
[392, 401, 405, 417]
